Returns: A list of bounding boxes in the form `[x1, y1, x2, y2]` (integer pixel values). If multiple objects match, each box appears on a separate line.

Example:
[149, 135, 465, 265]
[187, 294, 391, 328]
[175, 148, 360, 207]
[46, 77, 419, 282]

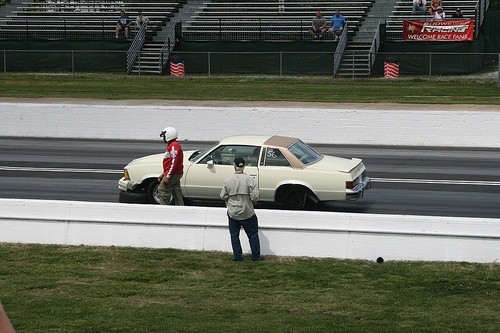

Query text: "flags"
[170, 56, 185, 76]
[384, 57, 400, 78]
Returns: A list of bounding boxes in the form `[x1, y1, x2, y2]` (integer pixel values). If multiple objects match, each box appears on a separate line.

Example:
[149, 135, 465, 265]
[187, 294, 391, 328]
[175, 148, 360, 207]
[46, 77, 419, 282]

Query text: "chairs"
[245, 146, 261, 165]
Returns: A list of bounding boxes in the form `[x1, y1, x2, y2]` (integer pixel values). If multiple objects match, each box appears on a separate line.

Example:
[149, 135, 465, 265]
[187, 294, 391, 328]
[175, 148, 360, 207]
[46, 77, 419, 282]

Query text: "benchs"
[0, 0, 491, 42]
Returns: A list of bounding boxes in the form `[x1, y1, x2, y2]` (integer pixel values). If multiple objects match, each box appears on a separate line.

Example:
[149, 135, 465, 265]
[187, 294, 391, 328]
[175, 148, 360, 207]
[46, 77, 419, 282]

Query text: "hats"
[234, 158, 245, 167]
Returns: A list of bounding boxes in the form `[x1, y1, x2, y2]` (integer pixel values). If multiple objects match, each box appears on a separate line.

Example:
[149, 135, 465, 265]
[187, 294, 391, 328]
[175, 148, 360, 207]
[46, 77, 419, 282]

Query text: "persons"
[451, 7, 464, 18]
[412, 0, 445, 19]
[328, 10, 347, 41]
[157, 127, 185, 206]
[0, 303, 17, 333]
[220, 158, 265, 261]
[136, 11, 150, 30]
[309, 11, 327, 39]
[115, 10, 132, 39]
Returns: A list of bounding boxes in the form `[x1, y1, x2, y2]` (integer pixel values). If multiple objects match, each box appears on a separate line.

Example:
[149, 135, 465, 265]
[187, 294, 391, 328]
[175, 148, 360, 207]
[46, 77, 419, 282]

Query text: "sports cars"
[116, 133, 372, 209]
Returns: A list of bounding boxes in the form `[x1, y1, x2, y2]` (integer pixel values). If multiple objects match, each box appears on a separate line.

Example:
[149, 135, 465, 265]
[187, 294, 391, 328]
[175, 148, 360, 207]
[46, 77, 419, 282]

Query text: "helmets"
[160, 127, 178, 142]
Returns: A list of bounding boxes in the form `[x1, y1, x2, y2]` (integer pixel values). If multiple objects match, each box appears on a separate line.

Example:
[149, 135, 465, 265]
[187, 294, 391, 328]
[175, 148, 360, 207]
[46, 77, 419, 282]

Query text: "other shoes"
[251, 258, 265, 261]
[234, 258, 243, 261]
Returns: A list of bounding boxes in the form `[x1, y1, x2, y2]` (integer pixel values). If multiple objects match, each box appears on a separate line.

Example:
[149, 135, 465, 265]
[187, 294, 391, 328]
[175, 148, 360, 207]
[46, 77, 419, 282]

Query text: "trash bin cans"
[383, 56, 400, 77]
[171, 55, 185, 76]
